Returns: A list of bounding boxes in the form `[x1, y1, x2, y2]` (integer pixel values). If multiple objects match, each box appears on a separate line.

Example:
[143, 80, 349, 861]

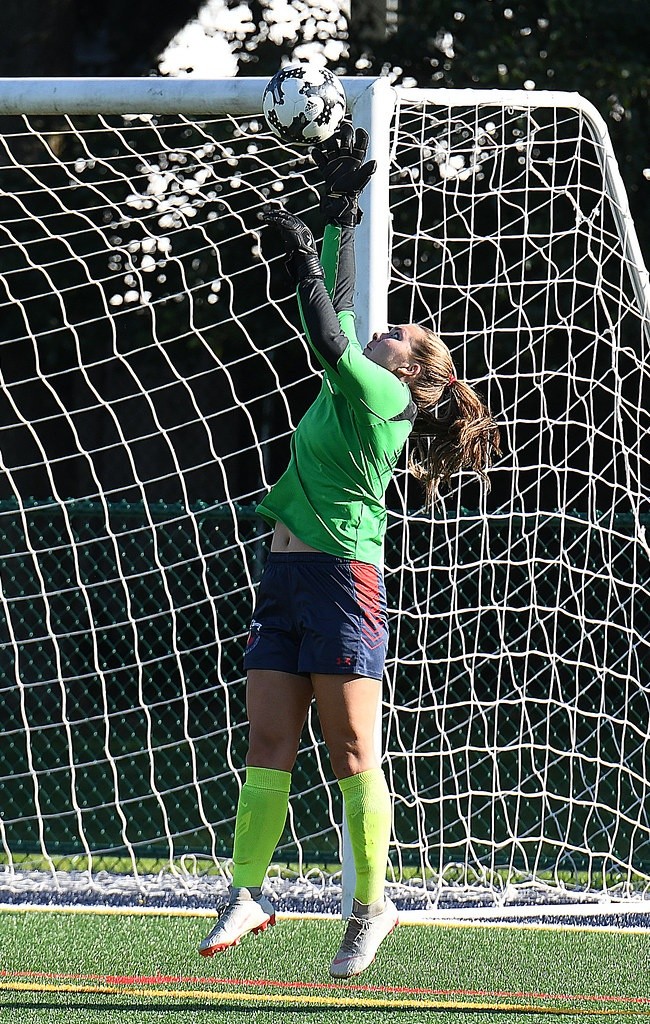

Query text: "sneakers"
[199, 895, 276, 958]
[328, 896, 399, 979]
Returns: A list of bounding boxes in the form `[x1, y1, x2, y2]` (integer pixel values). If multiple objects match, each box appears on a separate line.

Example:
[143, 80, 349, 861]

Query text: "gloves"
[262, 209, 326, 283]
[311, 123, 377, 230]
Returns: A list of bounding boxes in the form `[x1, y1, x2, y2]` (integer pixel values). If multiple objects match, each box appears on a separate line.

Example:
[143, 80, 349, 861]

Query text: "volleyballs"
[262, 61, 347, 146]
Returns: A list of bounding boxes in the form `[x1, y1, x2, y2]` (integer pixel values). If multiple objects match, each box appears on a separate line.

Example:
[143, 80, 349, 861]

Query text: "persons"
[200, 123, 501, 982]
